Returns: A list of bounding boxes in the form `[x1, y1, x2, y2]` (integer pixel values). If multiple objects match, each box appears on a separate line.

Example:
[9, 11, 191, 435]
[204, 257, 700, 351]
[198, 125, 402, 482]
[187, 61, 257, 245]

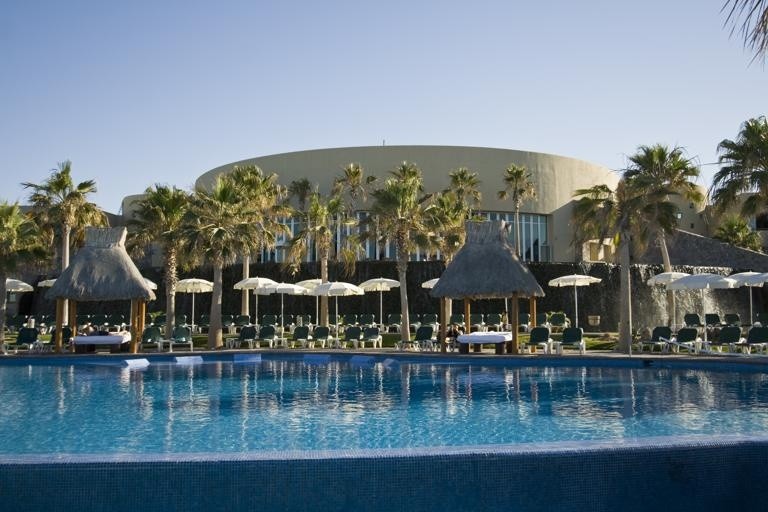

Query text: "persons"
[501, 311, 508, 330]
[445, 324, 464, 350]
[81, 324, 114, 336]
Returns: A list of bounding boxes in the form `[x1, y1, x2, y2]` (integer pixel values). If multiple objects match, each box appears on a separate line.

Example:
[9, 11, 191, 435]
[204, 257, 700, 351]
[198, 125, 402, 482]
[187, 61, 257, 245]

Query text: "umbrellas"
[647, 270, 768, 341]
[2, 278, 34, 329]
[233, 276, 401, 339]
[547, 274, 603, 329]
[129, 278, 157, 326]
[176, 276, 214, 333]
[421, 278, 441, 289]
[38, 279, 58, 288]
